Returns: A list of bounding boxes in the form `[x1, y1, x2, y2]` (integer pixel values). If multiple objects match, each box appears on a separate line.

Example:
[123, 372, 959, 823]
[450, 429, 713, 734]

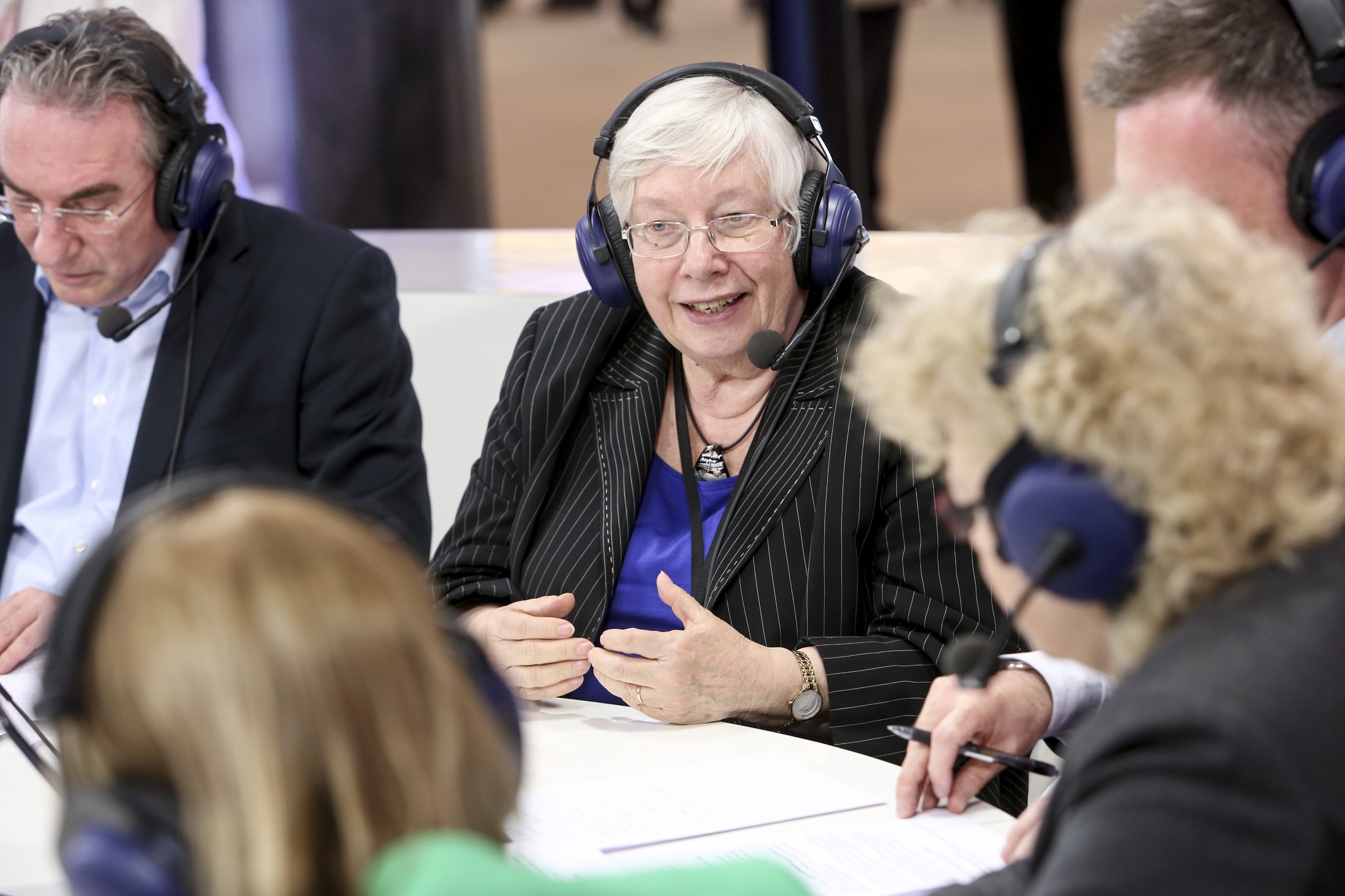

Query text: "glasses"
[620, 209, 790, 259]
[931, 492, 991, 538]
[0, 167, 169, 236]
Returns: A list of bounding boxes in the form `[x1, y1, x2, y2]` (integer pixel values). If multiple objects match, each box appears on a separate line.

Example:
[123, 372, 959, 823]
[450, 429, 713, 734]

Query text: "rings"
[636, 685, 645, 706]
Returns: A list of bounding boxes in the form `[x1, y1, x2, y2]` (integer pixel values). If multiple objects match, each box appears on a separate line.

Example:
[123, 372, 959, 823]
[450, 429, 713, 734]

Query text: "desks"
[506, 697, 1037, 896]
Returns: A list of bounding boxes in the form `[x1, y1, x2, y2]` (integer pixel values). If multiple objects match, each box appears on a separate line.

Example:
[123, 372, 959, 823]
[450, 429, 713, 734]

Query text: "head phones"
[575, 62, 869, 313]
[31, 465, 525, 896]
[1285, 0, 1345, 252]
[978, 232, 1154, 613]
[0, 24, 236, 233]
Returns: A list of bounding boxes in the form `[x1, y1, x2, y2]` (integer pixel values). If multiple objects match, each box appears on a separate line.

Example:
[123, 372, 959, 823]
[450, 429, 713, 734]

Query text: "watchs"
[999, 660, 1034, 671]
[733, 649, 823, 734]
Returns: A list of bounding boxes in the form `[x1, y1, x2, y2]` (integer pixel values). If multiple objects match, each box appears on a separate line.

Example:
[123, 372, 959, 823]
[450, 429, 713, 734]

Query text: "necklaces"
[682, 380, 778, 482]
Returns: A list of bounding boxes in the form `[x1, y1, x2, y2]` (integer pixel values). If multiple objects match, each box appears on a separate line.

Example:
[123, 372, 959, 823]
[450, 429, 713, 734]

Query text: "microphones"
[747, 241, 860, 372]
[97, 216, 222, 342]
[946, 577, 1039, 690]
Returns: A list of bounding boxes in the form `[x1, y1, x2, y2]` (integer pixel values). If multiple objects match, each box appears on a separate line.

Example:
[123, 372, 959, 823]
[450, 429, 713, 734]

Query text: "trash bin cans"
[886, 725, 1059, 777]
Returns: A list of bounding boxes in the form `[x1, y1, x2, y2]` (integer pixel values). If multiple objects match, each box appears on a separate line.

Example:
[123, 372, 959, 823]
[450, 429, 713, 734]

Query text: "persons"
[839, 179, 1345, 896]
[894, 0, 1345, 866]
[426, 77, 1032, 823]
[57, 487, 824, 896]
[0, 10, 439, 675]
